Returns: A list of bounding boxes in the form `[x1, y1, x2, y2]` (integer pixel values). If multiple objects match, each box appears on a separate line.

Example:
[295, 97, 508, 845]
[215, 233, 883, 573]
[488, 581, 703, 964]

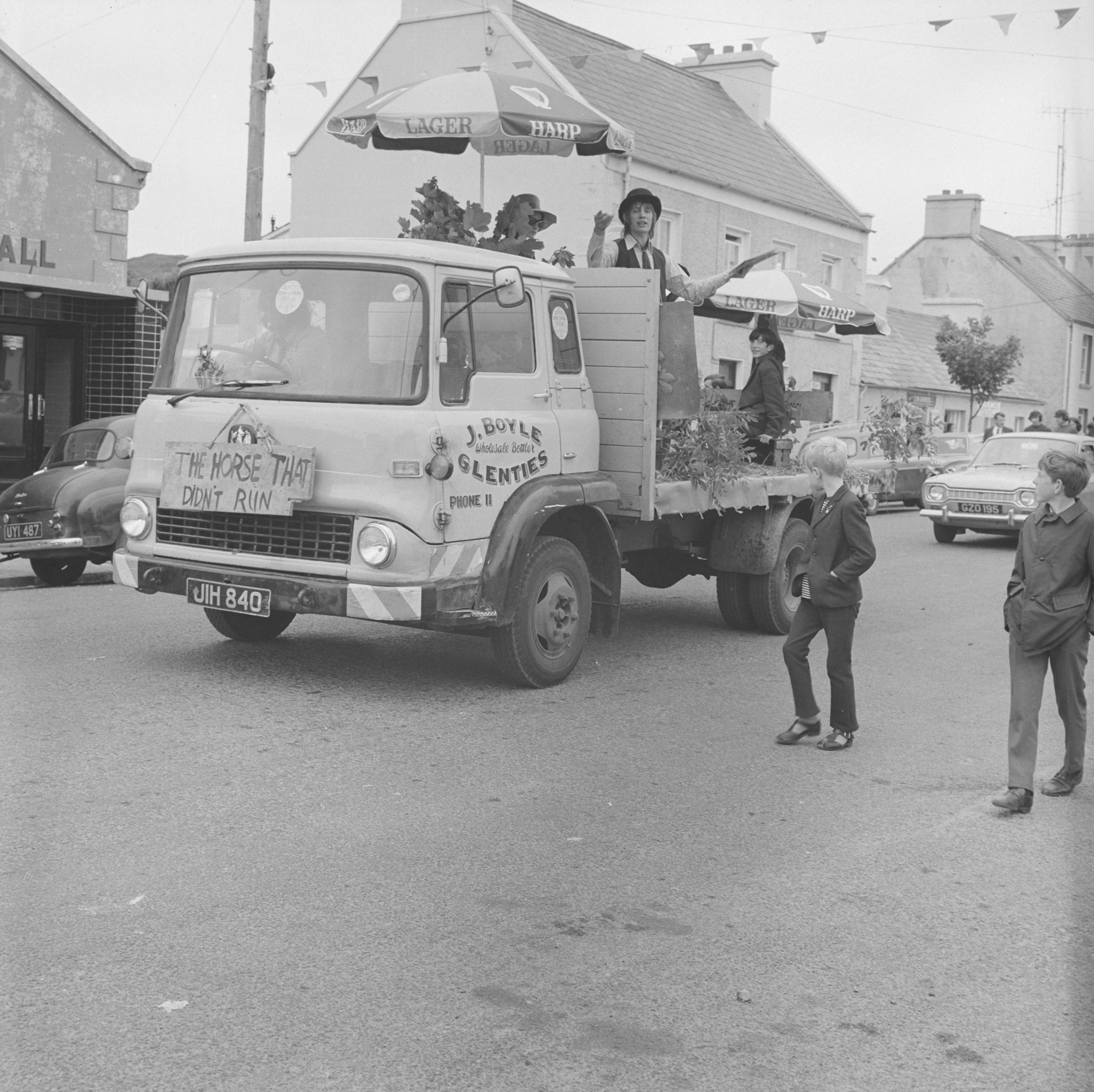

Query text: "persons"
[703, 374, 727, 389]
[988, 448, 1094, 812]
[195, 282, 330, 391]
[585, 188, 739, 306]
[1069, 416, 1084, 436]
[484, 194, 557, 256]
[777, 435, 878, 752]
[1055, 408, 1078, 434]
[1023, 410, 1052, 432]
[982, 413, 1014, 443]
[944, 422, 956, 433]
[734, 322, 786, 465]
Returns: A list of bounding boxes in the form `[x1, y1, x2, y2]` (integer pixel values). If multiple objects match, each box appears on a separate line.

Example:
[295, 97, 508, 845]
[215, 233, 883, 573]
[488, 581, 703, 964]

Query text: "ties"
[641, 250, 652, 270]
[996, 428, 999, 434]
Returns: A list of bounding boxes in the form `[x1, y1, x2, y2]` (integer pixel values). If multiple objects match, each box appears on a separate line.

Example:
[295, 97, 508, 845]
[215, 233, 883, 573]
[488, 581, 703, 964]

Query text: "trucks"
[111, 236, 838, 689]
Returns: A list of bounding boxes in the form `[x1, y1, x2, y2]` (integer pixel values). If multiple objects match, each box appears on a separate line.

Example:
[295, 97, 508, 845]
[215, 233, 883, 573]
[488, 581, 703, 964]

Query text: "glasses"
[528, 216, 547, 229]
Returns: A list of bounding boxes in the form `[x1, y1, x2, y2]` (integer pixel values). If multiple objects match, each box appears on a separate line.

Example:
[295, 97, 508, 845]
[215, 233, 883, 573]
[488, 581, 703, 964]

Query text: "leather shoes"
[991, 787, 1034, 813]
[1041, 765, 1084, 795]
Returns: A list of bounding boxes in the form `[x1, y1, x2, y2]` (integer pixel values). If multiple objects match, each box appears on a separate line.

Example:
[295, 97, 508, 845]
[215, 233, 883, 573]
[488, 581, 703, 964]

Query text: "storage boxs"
[743, 433, 794, 470]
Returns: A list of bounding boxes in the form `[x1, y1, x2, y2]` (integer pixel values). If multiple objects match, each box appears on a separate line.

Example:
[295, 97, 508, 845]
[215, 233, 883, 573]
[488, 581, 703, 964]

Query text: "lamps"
[22, 286, 44, 300]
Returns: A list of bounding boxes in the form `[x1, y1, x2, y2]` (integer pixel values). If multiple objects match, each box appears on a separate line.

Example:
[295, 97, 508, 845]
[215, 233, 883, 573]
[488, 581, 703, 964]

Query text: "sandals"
[776, 719, 820, 744]
[816, 729, 854, 750]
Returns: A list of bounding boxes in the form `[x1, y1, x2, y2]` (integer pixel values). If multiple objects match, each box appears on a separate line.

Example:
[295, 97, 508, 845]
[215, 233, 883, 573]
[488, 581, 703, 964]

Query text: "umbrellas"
[326, 59, 633, 209]
[692, 261, 892, 337]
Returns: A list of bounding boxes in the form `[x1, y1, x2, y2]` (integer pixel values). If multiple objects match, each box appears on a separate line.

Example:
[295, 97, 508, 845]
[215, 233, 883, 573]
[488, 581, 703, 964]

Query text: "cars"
[794, 420, 948, 515]
[927, 430, 989, 474]
[921, 430, 1094, 542]
[0, 414, 140, 587]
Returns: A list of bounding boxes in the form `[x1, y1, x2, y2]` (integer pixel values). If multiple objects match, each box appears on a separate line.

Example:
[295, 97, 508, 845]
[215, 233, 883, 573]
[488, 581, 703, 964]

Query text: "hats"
[618, 187, 661, 225]
[514, 194, 557, 232]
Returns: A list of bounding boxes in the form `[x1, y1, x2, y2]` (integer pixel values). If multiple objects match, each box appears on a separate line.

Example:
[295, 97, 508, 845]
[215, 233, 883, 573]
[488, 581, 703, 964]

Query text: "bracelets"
[724, 270, 731, 283]
[500, 240, 506, 249]
